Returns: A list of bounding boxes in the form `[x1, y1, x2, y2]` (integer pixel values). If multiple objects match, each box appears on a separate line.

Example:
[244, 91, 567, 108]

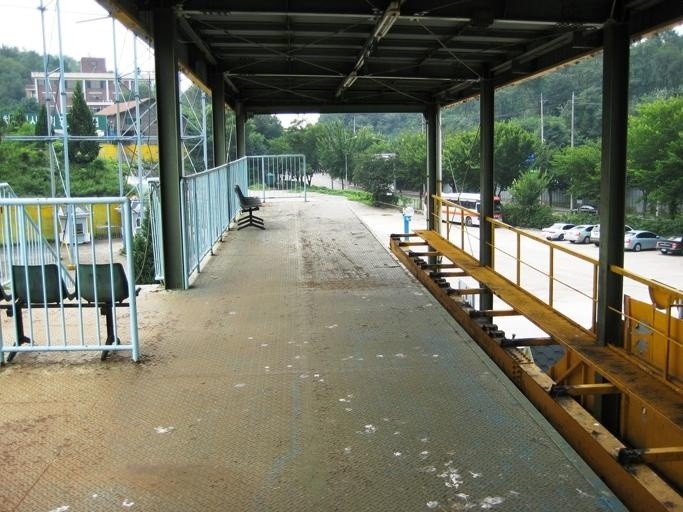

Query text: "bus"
[423, 191, 504, 227]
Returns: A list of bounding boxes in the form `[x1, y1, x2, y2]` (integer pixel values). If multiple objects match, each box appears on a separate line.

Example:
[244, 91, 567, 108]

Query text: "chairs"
[0, 263, 141, 361]
[234, 184, 265, 230]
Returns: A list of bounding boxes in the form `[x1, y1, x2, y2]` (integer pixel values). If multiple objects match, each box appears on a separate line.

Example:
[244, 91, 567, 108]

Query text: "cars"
[570, 205, 599, 215]
[541, 223, 683, 254]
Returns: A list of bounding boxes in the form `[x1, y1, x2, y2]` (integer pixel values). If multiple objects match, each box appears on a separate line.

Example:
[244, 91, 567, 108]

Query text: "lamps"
[340, 2, 400, 89]
